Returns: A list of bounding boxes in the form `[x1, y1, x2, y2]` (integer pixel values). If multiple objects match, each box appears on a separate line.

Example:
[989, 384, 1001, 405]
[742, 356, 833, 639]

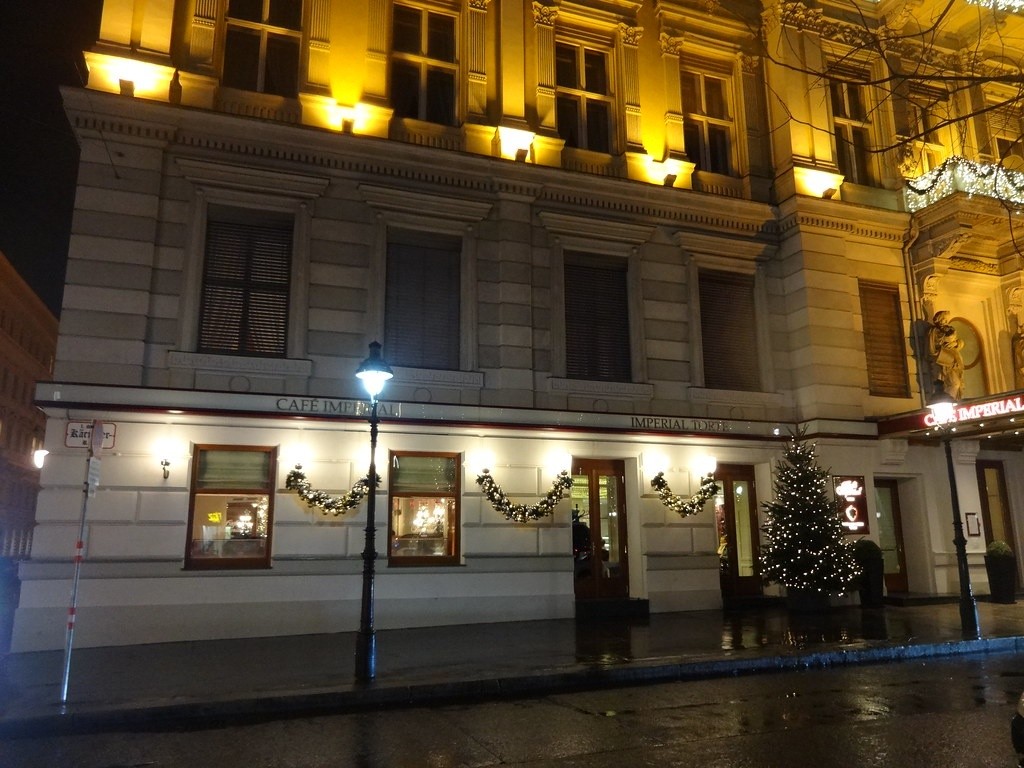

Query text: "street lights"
[350, 342, 396, 680]
[925, 377, 985, 641]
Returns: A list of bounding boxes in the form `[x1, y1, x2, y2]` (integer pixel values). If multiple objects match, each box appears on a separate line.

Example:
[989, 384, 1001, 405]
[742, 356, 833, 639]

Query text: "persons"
[923, 311, 965, 401]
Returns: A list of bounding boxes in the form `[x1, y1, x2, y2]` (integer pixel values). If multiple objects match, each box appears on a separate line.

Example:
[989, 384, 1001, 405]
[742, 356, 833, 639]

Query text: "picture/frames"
[965, 512, 981, 537]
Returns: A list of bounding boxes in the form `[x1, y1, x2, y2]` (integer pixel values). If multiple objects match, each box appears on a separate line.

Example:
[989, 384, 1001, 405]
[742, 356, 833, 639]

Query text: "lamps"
[515, 148, 529, 163]
[341, 117, 354, 133]
[664, 174, 677, 187]
[154, 442, 173, 479]
[822, 188, 837, 199]
[119, 78, 135, 97]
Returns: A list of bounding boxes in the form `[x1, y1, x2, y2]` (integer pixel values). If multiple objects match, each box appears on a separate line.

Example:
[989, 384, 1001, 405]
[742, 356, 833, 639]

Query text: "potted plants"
[984, 539, 1017, 604]
[850, 539, 884, 609]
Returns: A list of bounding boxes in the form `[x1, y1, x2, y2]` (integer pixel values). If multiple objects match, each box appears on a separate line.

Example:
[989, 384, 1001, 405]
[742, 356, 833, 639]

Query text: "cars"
[1010, 687, 1024, 768]
